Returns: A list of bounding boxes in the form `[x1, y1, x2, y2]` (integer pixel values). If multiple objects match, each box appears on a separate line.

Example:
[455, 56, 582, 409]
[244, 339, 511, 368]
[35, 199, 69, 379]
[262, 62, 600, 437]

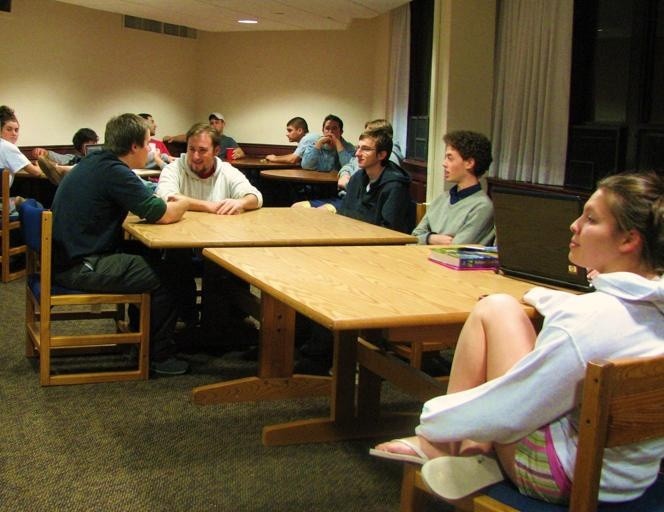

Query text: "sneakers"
[149, 358, 189, 374]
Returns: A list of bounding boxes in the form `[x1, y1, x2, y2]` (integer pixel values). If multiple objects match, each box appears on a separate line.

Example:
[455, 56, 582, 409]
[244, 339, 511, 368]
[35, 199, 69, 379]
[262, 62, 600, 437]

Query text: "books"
[429, 248, 498, 268]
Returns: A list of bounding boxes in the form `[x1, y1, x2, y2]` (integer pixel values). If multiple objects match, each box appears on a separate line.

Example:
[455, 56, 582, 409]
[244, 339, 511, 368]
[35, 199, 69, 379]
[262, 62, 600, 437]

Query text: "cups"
[225, 148, 234, 161]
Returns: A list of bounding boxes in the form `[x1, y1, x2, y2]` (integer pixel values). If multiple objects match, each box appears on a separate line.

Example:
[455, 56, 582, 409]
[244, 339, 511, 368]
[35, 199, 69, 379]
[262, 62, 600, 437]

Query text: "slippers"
[421, 454, 506, 501]
[369, 439, 428, 465]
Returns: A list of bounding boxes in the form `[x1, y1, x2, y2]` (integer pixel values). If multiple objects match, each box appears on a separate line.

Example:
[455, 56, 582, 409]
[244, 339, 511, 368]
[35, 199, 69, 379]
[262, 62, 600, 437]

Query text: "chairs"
[2, 168, 27, 283]
[413, 203, 426, 227]
[16, 199, 151, 386]
[400, 354, 664, 511]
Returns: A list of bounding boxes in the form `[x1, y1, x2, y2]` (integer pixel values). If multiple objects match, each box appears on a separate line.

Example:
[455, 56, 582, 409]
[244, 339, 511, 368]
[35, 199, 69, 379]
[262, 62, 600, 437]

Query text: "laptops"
[490, 186, 596, 293]
[85, 144, 105, 156]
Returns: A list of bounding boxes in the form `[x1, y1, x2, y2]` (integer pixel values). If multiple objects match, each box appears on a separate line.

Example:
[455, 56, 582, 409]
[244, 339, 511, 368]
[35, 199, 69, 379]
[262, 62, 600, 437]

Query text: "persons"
[369, 172, 664, 506]
[359, 129, 493, 426]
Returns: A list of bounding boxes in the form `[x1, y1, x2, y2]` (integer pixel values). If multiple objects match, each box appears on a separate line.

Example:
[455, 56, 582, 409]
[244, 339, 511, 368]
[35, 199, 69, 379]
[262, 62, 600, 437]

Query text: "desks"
[1, 149, 428, 208]
[120, 207, 420, 356]
[202, 243, 545, 445]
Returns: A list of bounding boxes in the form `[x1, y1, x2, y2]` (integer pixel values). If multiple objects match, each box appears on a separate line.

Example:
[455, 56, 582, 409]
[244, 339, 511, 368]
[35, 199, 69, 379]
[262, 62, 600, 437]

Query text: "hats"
[209, 112, 224, 122]
